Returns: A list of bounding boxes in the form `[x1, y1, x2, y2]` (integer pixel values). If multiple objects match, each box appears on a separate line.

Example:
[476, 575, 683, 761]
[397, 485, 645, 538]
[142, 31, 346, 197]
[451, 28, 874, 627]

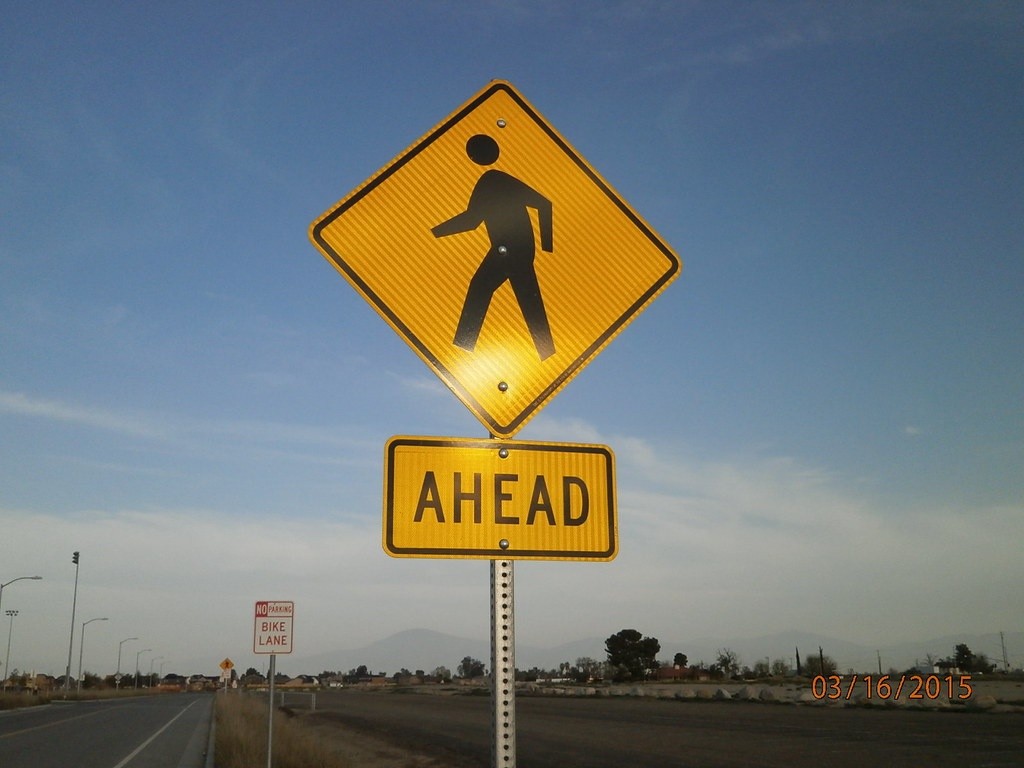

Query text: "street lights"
[2, 610, 19, 693]
[116, 637, 172, 691]
[0, 576, 43, 609]
[77, 617, 108, 691]
[64, 551, 79, 696]
